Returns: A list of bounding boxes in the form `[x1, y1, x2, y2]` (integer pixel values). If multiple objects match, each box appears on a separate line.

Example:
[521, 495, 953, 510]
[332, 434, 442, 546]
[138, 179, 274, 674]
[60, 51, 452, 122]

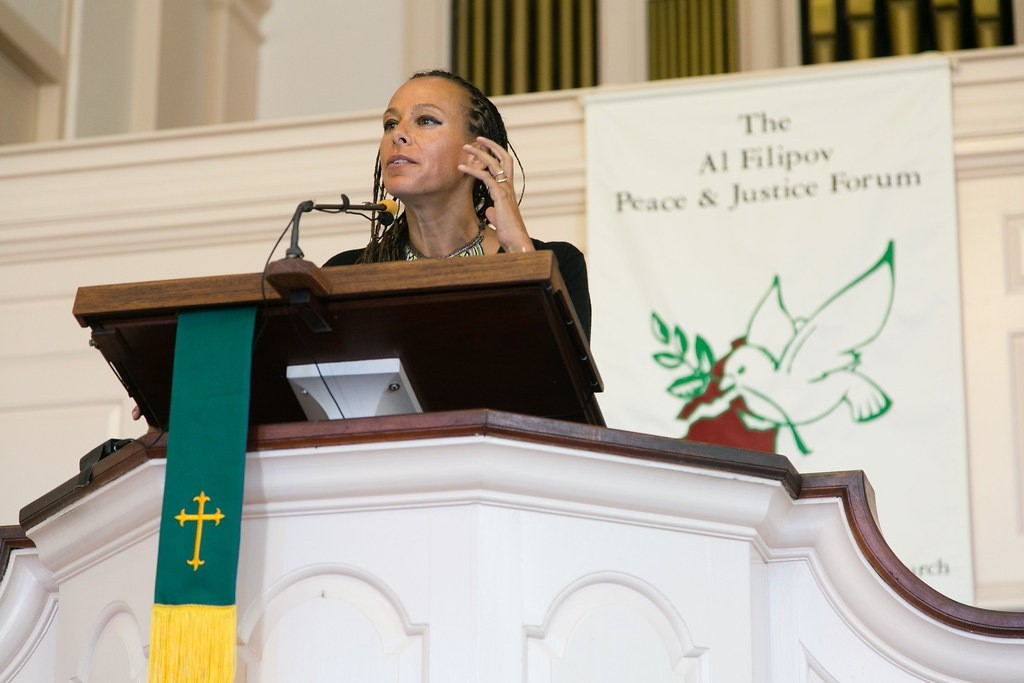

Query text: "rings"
[498, 176, 507, 183]
[493, 170, 504, 178]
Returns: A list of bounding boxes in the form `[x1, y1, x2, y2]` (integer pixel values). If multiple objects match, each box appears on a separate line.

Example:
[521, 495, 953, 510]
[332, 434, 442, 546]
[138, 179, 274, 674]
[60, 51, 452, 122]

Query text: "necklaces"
[402, 217, 485, 261]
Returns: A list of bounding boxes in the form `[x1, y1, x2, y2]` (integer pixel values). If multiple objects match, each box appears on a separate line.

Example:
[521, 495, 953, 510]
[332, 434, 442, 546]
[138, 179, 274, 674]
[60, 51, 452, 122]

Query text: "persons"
[131, 70, 592, 420]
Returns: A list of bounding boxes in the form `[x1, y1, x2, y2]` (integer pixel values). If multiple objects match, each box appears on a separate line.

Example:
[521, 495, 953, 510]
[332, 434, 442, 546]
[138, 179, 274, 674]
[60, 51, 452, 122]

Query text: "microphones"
[286, 200, 398, 260]
[377, 212, 394, 225]
